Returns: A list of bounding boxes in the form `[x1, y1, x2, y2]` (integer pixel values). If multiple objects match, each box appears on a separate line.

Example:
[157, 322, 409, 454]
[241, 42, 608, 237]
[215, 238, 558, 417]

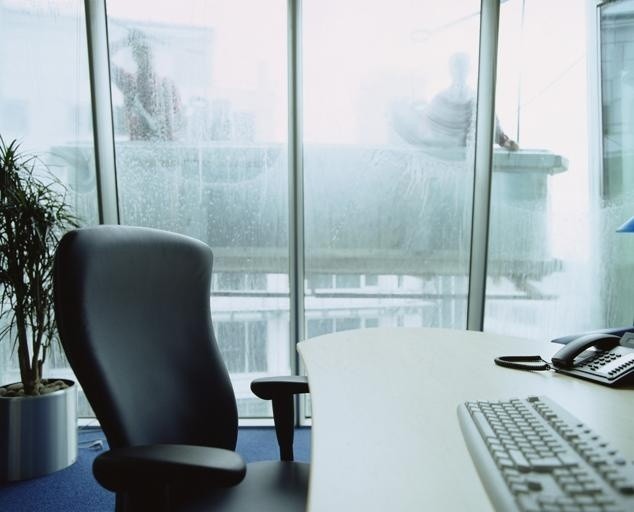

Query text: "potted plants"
[0, 134, 84, 485]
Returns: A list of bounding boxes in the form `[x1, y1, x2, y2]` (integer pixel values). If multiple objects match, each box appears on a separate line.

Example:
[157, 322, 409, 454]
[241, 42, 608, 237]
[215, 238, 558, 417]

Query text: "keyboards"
[457, 394, 634, 512]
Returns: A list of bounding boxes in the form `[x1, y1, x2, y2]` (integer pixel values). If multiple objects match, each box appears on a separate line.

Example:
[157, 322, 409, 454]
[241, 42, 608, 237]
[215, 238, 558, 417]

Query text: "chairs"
[51, 227, 309, 511]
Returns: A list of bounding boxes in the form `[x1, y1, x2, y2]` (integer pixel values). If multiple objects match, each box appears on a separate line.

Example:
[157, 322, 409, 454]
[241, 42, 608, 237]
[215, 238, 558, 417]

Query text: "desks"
[296, 326, 632, 512]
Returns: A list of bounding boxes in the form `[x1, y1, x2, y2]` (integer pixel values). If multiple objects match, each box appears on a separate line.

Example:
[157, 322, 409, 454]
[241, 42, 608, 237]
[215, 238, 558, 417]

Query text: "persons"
[425, 55, 519, 154]
[106, 27, 190, 145]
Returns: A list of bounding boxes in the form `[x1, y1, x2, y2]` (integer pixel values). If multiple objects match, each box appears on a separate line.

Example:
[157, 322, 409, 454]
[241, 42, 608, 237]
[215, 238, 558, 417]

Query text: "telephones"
[546, 333, 634, 387]
